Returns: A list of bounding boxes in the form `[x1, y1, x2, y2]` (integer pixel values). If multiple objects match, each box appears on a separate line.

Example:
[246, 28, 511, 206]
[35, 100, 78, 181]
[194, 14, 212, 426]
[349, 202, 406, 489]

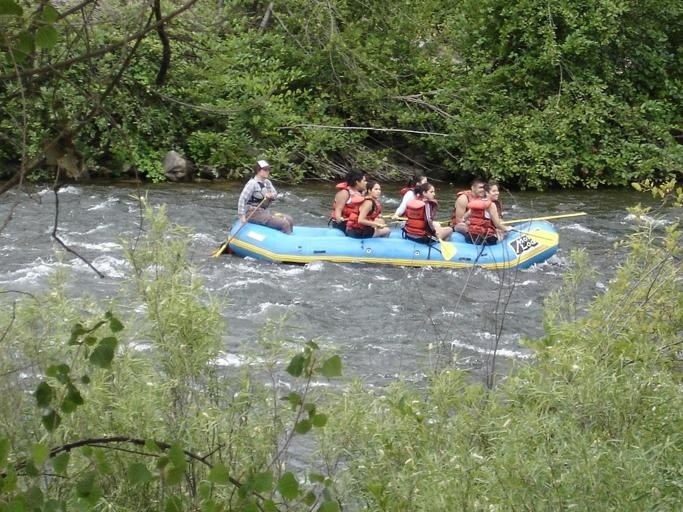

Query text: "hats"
[255, 160, 269, 172]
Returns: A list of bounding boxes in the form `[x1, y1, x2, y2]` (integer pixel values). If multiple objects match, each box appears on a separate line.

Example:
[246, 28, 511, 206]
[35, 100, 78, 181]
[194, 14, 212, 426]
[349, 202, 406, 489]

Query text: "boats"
[214, 216, 559, 272]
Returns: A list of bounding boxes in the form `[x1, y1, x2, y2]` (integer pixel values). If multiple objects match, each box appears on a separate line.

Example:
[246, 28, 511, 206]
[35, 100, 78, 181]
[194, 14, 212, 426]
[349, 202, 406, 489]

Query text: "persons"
[450, 178, 488, 234]
[345, 181, 391, 238]
[390, 176, 429, 228]
[462, 182, 512, 245]
[237, 158, 293, 234]
[331, 170, 368, 232]
[401, 182, 453, 244]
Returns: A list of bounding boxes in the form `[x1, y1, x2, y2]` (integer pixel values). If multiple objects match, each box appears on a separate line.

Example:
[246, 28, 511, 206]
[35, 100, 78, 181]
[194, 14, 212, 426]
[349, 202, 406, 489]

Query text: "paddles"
[212, 197, 267, 258]
[438, 237, 457, 260]
[511, 229, 559, 247]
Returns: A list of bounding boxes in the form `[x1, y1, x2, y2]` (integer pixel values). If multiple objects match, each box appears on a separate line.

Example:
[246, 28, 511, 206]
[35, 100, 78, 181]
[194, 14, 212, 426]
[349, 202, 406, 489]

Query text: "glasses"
[261, 167, 270, 171]
